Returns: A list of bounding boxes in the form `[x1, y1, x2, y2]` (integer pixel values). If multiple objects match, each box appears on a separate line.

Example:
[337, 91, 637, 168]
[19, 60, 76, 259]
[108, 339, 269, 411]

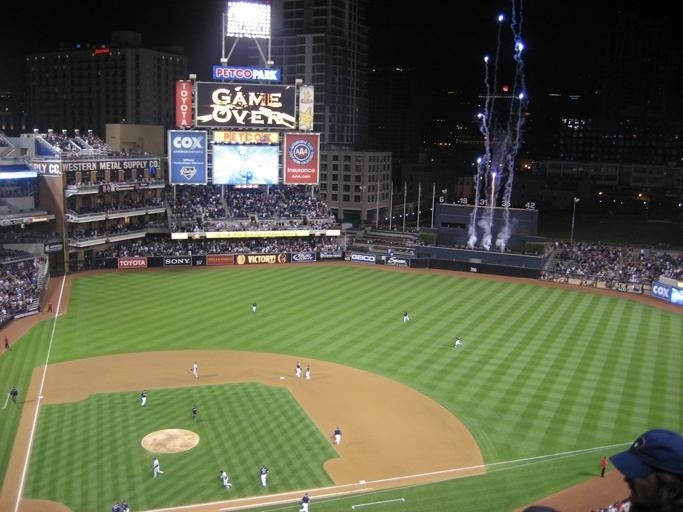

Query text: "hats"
[606, 428, 683, 478]
